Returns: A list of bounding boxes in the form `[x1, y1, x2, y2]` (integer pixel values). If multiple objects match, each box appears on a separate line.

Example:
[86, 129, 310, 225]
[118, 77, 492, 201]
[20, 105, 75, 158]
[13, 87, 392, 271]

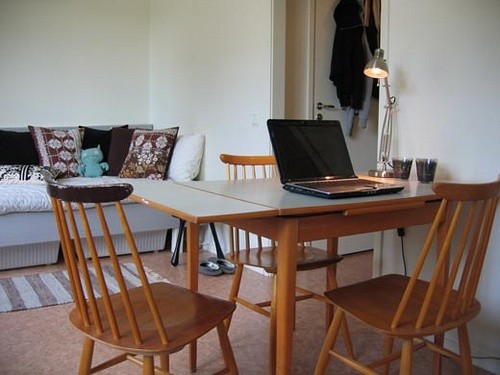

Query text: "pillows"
[119, 127, 179, 180]
[108, 127, 134, 177]
[0, 162, 58, 187]
[0, 131, 39, 165]
[79, 125, 128, 176]
[29, 123, 87, 179]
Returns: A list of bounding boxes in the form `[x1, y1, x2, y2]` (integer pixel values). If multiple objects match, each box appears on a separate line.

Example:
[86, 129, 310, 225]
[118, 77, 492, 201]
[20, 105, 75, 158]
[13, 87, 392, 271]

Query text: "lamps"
[363, 45, 397, 179]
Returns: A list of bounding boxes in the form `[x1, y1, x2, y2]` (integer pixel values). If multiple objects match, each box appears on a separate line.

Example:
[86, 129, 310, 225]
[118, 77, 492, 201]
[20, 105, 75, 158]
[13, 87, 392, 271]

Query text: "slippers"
[198, 260, 223, 276]
[209, 256, 236, 274]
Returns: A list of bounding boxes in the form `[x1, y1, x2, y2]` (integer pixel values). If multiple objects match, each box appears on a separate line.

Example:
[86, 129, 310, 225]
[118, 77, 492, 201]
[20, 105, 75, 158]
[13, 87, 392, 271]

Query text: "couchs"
[0, 122, 205, 255]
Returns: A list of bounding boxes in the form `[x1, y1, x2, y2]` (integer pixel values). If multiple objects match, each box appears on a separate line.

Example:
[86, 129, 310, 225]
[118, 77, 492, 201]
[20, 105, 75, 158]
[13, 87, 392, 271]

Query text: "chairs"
[41, 178, 241, 375]
[310, 181, 499, 375]
[219, 152, 358, 375]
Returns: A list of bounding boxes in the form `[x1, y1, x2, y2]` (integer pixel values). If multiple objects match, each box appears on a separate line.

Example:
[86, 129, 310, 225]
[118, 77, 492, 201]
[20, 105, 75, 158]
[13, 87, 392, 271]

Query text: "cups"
[390, 157, 414, 181]
[416, 158, 438, 184]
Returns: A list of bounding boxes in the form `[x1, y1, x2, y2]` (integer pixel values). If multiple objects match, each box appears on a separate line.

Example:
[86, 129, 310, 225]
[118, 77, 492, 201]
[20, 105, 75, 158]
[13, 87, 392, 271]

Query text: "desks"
[125, 172, 451, 375]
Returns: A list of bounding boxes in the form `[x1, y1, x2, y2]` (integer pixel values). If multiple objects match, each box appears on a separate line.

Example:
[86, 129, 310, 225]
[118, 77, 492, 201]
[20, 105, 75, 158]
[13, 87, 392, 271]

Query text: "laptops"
[267, 119, 405, 199]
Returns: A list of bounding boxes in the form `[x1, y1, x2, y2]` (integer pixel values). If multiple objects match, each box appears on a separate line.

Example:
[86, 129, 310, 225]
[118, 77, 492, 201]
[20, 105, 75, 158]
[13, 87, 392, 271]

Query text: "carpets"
[0, 262, 175, 313]
